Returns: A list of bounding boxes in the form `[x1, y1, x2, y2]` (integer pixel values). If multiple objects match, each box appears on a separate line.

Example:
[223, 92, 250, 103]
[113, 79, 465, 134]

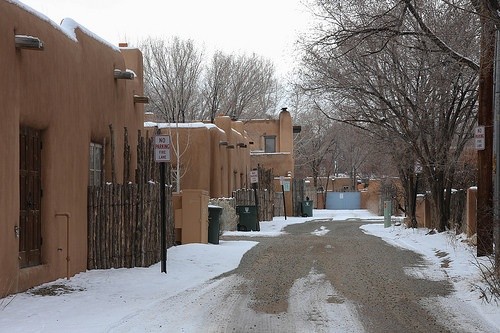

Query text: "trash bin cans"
[236, 205, 259, 231]
[301, 200, 313, 217]
[209, 206, 223, 244]
